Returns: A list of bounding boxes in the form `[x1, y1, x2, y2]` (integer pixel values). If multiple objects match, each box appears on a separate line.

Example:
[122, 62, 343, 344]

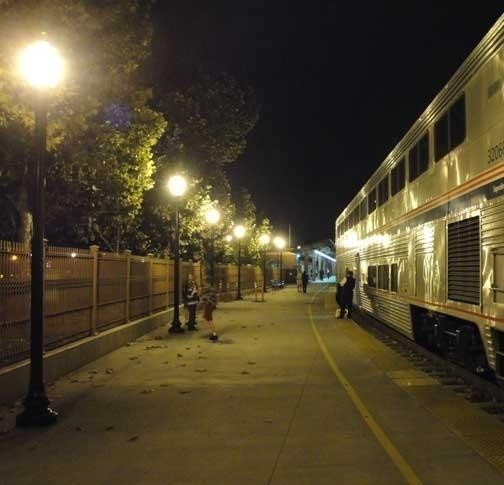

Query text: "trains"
[335, 11, 503, 389]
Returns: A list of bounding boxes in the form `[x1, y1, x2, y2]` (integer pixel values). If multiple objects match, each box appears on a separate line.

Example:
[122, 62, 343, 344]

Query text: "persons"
[336, 271, 356, 320]
[296, 268, 302, 293]
[318, 268, 325, 281]
[183, 279, 200, 331]
[198, 274, 218, 341]
[302, 270, 308, 293]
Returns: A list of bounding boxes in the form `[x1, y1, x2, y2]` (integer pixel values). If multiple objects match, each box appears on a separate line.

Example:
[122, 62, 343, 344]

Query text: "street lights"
[232, 223, 245, 302]
[257, 231, 270, 293]
[165, 173, 188, 335]
[276, 236, 285, 288]
[205, 204, 219, 286]
[8, 26, 69, 428]
